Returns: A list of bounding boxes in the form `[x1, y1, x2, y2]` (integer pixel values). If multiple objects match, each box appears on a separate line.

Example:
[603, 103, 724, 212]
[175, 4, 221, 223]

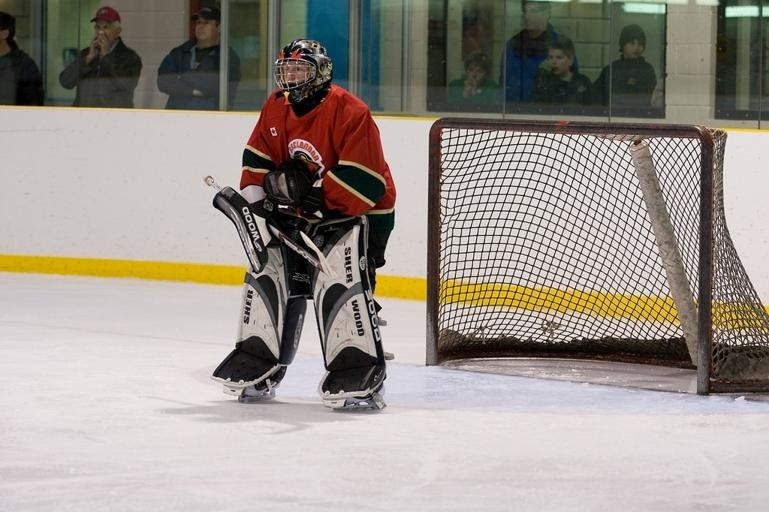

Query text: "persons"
[155, 7, 242, 110]
[500, 1, 578, 113]
[524, 34, 595, 118]
[448, 52, 500, 114]
[59, 7, 143, 108]
[203, 38, 398, 411]
[593, 24, 656, 116]
[0, 12, 44, 106]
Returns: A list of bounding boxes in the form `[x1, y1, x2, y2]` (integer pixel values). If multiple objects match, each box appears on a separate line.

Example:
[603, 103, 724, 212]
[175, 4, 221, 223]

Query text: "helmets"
[273, 38, 334, 104]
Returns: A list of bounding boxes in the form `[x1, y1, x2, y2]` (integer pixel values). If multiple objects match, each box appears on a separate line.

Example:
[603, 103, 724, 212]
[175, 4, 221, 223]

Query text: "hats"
[618, 23, 646, 48]
[190, 5, 219, 21]
[90, 6, 120, 23]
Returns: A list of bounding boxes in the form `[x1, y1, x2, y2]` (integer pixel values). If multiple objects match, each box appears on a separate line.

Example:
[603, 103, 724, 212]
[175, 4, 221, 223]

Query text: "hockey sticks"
[204, 174, 339, 282]
